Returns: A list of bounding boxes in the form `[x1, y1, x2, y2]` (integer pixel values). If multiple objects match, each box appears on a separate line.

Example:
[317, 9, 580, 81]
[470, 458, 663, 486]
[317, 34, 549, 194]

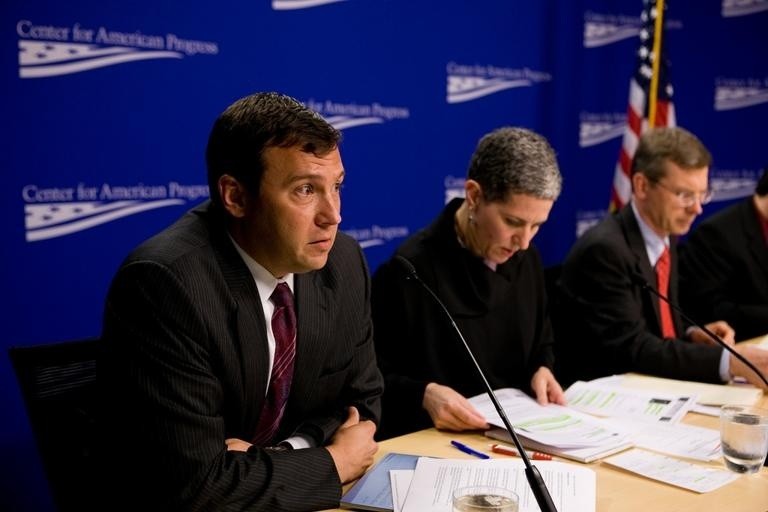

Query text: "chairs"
[5, 339, 122, 512]
[545, 265, 575, 338]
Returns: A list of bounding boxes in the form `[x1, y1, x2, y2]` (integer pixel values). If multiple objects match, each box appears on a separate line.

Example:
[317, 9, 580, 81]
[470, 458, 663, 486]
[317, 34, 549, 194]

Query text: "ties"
[253, 282, 297, 446]
[656, 244, 676, 339]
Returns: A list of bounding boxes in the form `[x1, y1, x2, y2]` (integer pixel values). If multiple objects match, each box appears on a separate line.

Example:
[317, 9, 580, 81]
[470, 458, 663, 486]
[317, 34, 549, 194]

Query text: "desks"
[320, 334, 766, 512]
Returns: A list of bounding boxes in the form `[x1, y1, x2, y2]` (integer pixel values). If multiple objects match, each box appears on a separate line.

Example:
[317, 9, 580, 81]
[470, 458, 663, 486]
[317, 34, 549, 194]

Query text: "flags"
[604, 1, 681, 217]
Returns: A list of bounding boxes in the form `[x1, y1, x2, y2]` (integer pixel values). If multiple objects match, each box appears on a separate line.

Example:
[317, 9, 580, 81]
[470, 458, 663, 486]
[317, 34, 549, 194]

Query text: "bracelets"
[265, 444, 287, 453]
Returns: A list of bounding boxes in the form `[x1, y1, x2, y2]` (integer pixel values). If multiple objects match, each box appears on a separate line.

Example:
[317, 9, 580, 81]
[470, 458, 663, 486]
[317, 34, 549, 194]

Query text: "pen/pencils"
[451, 440, 489, 459]
[492, 444, 552, 460]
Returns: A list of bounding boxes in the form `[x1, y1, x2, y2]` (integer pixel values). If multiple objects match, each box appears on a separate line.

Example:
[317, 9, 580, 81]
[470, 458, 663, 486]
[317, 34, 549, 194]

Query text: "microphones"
[393, 253, 558, 512]
[628, 271, 768, 386]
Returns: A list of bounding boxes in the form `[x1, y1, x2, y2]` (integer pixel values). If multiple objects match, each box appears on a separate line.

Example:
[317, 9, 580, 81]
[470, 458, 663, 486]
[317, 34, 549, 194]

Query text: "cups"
[451, 485, 520, 512]
[719, 403, 768, 477]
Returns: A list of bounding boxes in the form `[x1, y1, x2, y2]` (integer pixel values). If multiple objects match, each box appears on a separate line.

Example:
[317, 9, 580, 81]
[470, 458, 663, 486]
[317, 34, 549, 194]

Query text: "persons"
[675, 167, 768, 348]
[368, 125, 570, 443]
[70, 90, 390, 510]
[548, 123, 767, 398]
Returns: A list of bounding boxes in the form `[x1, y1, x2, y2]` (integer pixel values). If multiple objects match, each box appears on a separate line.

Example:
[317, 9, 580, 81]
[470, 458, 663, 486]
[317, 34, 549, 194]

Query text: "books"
[486, 427, 637, 465]
[339, 452, 448, 511]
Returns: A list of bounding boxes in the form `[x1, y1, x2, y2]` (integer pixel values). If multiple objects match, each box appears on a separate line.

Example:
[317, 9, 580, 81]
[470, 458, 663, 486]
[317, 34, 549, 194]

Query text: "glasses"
[646, 176, 714, 206]
